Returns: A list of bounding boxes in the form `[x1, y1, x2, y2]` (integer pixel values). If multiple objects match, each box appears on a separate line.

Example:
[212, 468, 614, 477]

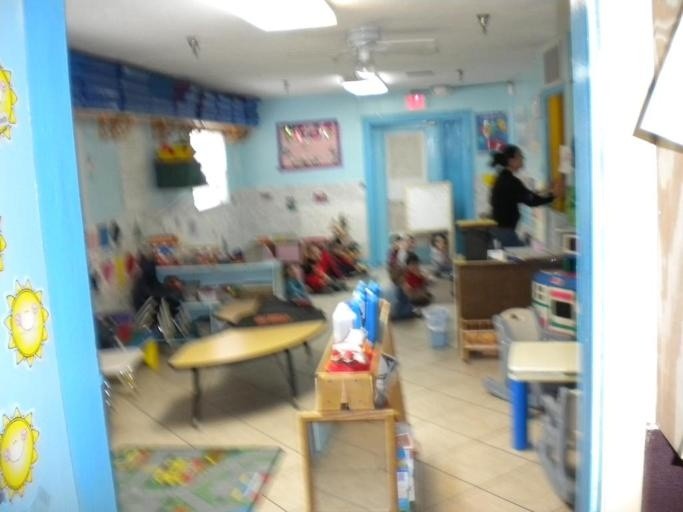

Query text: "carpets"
[110, 448, 281, 511]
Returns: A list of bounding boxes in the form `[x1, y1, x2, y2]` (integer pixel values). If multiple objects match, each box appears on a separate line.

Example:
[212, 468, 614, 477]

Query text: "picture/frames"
[276, 120, 342, 171]
[473, 110, 509, 153]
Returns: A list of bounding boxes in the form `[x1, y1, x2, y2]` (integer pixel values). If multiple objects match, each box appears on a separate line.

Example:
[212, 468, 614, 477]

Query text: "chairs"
[96, 314, 147, 403]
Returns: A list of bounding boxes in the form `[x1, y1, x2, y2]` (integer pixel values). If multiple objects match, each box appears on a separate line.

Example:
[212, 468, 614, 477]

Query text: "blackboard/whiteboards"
[406, 181, 452, 234]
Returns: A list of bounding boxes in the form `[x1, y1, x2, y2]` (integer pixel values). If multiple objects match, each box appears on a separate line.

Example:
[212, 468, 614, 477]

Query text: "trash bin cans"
[423, 306, 449, 349]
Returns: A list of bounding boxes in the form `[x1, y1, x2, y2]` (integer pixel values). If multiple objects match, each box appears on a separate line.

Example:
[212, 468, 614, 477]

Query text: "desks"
[168, 320, 326, 425]
[506, 340, 579, 451]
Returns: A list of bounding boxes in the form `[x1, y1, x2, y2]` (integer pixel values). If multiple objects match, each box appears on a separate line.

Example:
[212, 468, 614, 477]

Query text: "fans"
[332, 23, 437, 64]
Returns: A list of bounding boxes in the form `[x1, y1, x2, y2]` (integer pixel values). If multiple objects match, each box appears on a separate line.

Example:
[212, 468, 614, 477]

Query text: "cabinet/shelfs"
[454, 253, 562, 360]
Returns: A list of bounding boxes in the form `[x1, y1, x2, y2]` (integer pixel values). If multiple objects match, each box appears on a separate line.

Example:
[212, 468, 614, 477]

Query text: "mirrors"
[298, 409, 399, 512]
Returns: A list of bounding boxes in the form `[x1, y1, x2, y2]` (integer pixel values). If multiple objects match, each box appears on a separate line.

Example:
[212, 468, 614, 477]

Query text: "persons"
[386, 228, 453, 316]
[490, 144, 563, 246]
[283, 233, 368, 301]
